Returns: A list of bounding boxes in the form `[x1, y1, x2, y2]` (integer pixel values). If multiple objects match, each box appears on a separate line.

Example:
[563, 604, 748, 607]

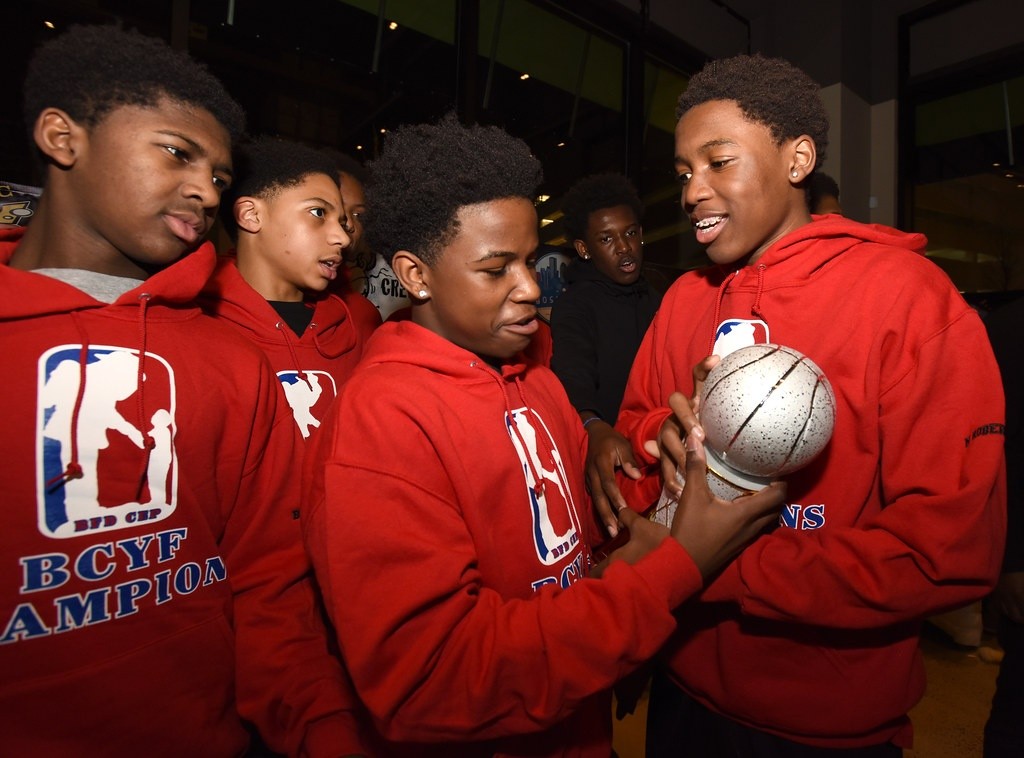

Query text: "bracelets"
[584, 417, 601, 427]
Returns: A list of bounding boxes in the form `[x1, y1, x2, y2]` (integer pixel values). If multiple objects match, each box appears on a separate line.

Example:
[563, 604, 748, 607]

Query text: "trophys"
[653, 344, 837, 529]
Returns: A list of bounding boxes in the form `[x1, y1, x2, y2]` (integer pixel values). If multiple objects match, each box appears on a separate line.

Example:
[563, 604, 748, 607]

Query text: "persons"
[805, 173, 841, 218]
[199, 136, 362, 440]
[551, 173, 663, 537]
[614, 55, 1006, 758]
[300, 116, 790, 757]
[321, 147, 382, 344]
[0, 28, 372, 756]
